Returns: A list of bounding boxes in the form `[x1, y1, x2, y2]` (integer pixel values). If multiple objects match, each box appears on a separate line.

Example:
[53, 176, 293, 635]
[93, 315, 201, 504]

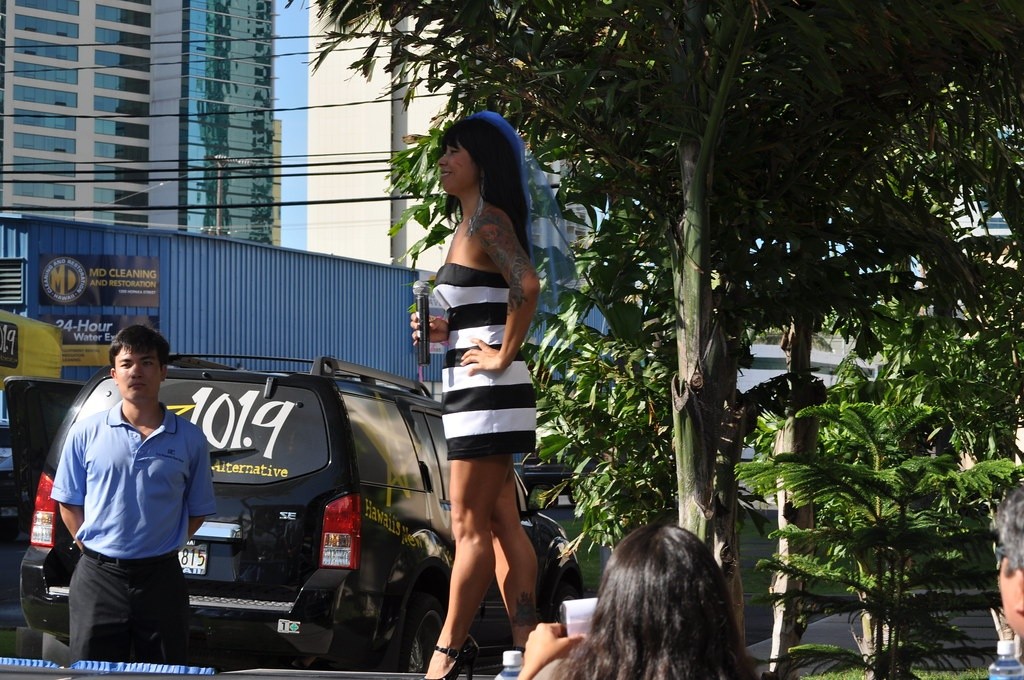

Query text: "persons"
[50, 324, 217, 667]
[994, 488, 1023, 641]
[516, 523, 759, 680]
[410, 112, 539, 680]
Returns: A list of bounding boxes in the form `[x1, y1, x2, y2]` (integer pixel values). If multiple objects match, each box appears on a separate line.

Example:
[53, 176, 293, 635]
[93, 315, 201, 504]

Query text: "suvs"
[4, 354, 585, 680]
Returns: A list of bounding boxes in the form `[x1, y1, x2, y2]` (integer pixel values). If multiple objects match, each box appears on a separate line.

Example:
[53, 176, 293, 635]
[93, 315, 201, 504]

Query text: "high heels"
[419, 634, 480, 680]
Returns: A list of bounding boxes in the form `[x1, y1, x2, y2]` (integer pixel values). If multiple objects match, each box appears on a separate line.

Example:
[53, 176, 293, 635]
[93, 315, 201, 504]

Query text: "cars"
[0, 418, 22, 545]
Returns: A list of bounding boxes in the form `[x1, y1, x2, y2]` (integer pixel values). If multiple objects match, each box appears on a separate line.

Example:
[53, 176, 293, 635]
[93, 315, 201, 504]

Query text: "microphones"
[412, 280, 431, 367]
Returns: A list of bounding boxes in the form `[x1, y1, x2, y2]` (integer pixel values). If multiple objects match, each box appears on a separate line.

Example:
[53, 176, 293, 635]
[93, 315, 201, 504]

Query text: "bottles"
[989, 640, 1024, 680]
[494, 650, 522, 680]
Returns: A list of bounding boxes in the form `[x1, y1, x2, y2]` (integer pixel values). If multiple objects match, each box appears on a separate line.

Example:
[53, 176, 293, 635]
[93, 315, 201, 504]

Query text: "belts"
[83, 545, 180, 565]
